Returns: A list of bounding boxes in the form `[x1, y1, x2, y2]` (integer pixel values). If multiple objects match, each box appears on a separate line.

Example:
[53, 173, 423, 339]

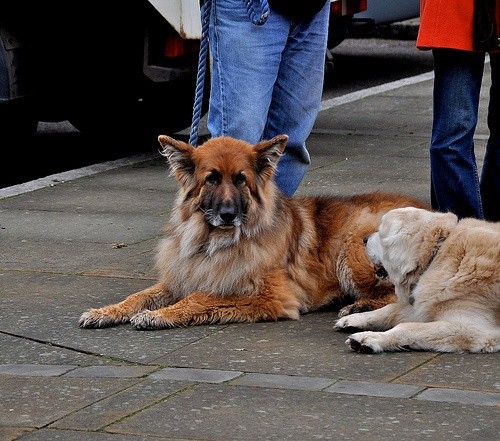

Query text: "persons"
[200, 0, 331, 196]
[415, 0, 500, 222]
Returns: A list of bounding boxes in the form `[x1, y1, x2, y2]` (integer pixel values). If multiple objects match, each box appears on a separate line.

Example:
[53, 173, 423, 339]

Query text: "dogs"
[78, 133, 500, 354]
